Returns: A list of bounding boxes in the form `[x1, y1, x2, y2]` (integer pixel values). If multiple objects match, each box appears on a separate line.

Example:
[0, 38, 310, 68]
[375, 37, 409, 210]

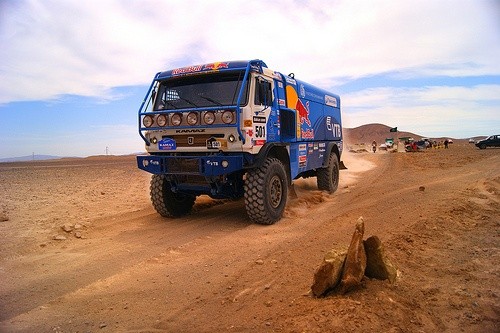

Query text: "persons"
[404, 137, 444, 151]
[372, 139, 377, 152]
[444, 139, 449, 149]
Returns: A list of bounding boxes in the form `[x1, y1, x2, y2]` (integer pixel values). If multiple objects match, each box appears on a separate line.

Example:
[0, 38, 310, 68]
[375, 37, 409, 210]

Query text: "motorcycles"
[372, 145, 377, 153]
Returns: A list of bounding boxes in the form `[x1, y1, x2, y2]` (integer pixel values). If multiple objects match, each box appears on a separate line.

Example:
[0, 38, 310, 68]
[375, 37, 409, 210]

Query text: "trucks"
[136, 59, 346, 226]
[385, 138, 393, 143]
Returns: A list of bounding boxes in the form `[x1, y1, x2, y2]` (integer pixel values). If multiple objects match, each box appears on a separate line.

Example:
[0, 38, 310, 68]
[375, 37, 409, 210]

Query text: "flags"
[389, 127, 397, 133]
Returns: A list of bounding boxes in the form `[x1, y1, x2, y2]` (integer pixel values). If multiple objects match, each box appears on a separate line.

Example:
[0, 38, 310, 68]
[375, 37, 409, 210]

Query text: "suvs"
[468, 139, 474, 143]
[475, 135, 500, 150]
[421, 138, 433, 147]
[448, 140, 453, 144]
